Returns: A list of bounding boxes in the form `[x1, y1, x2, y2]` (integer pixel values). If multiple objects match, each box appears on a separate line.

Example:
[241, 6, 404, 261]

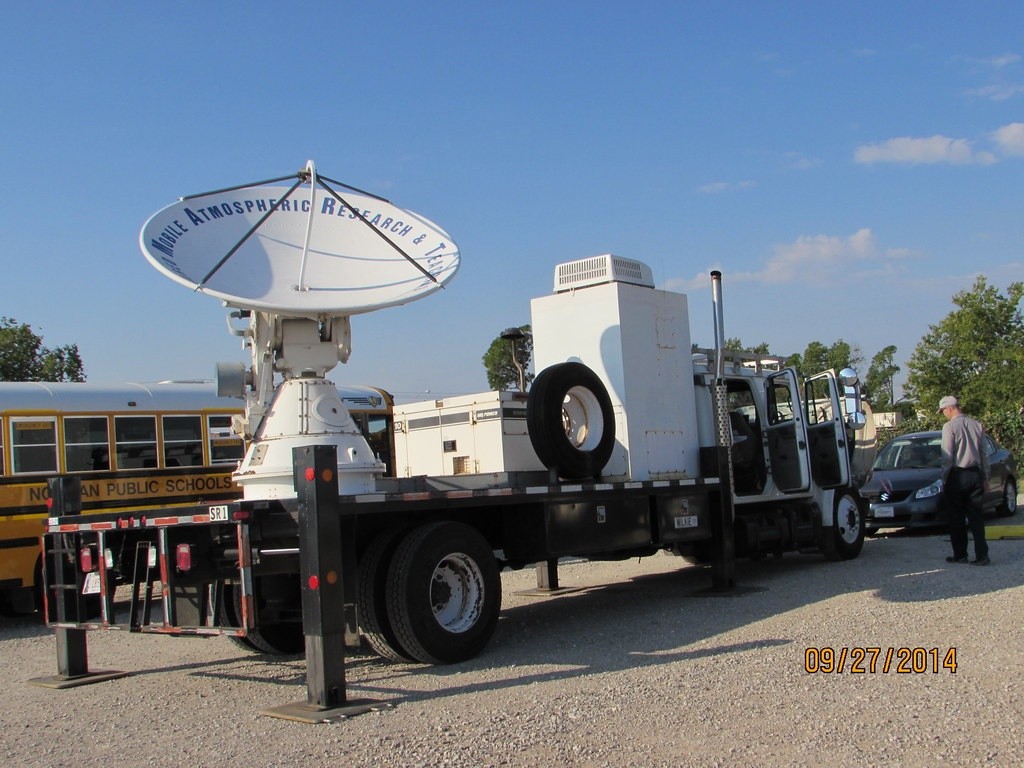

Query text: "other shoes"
[946, 556, 968, 564]
[971, 557, 990, 566]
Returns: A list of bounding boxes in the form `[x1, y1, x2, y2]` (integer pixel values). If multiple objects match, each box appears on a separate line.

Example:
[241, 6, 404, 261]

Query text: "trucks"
[27, 159, 869, 726]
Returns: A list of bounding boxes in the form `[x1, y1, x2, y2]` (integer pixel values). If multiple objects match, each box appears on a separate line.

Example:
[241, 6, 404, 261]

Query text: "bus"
[0, 380, 398, 622]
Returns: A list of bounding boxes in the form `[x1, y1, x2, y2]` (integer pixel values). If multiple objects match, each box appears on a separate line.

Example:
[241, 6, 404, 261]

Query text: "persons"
[927, 449, 942, 466]
[901, 446, 921, 466]
[935, 395, 991, 567]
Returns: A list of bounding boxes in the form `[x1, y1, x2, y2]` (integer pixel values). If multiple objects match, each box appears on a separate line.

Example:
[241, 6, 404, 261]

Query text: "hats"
[936, 396, 958, 414]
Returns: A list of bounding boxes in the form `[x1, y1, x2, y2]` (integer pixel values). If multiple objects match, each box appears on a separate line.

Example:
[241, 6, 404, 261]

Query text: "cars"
[858, 430, 1019, 536]
